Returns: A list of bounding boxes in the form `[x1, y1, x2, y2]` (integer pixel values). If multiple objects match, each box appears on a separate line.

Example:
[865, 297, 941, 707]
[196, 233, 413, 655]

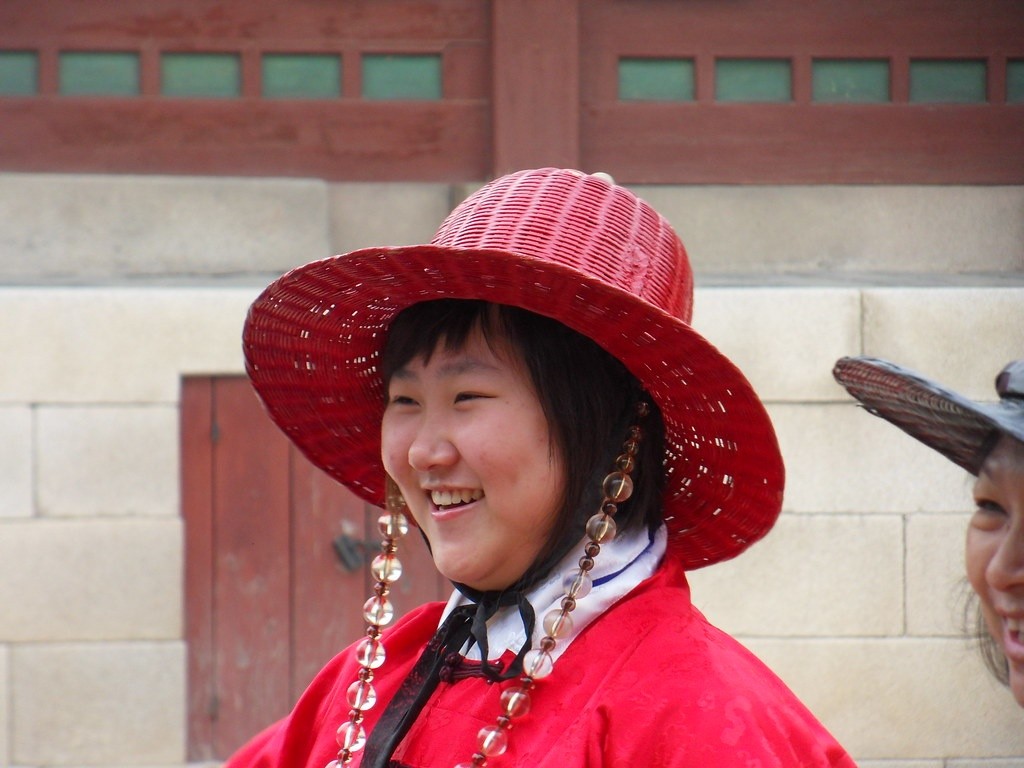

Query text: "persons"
[964, 357, 1023, 712]
[211, 165, 857, 768]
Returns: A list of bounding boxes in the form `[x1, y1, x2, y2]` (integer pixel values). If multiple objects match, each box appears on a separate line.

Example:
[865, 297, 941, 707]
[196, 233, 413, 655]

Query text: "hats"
[831, 356, 1024, 476]
[239, 167, 787, 572]
